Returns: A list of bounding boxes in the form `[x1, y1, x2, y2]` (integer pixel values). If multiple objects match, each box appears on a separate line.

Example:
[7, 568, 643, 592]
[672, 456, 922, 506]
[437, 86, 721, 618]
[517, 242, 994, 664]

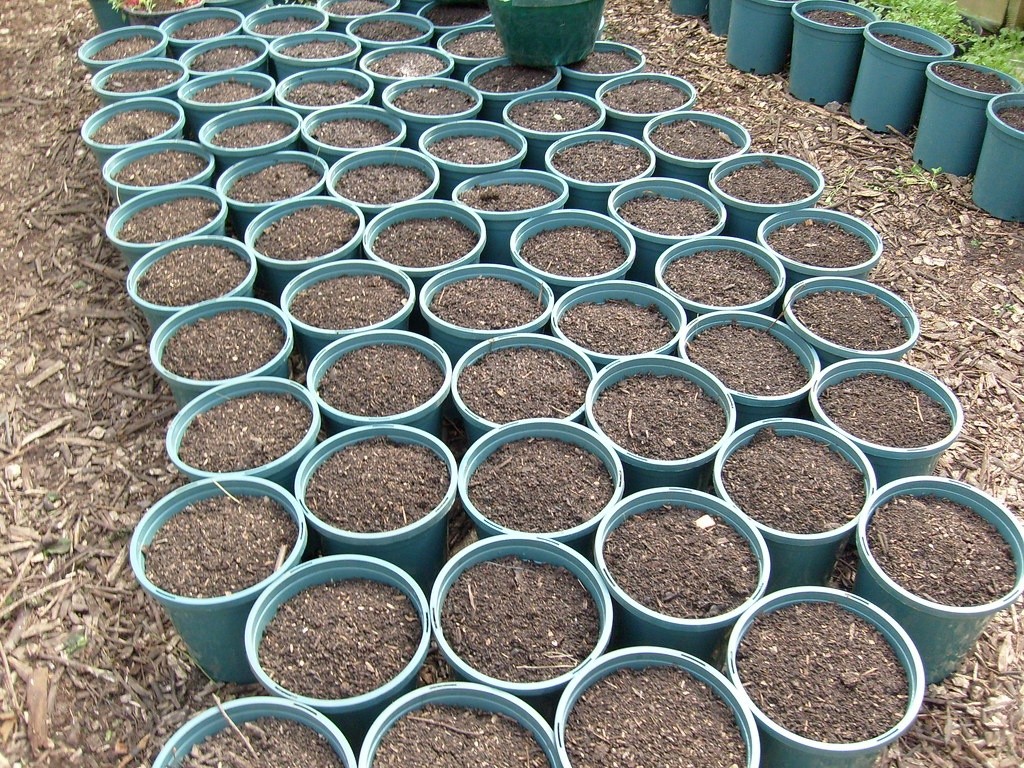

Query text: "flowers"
[109, 0, 205, 22]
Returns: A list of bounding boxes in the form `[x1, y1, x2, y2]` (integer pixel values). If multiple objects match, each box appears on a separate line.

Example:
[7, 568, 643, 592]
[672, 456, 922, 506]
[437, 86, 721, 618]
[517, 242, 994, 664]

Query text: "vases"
[76, 0, 1024, 768]
